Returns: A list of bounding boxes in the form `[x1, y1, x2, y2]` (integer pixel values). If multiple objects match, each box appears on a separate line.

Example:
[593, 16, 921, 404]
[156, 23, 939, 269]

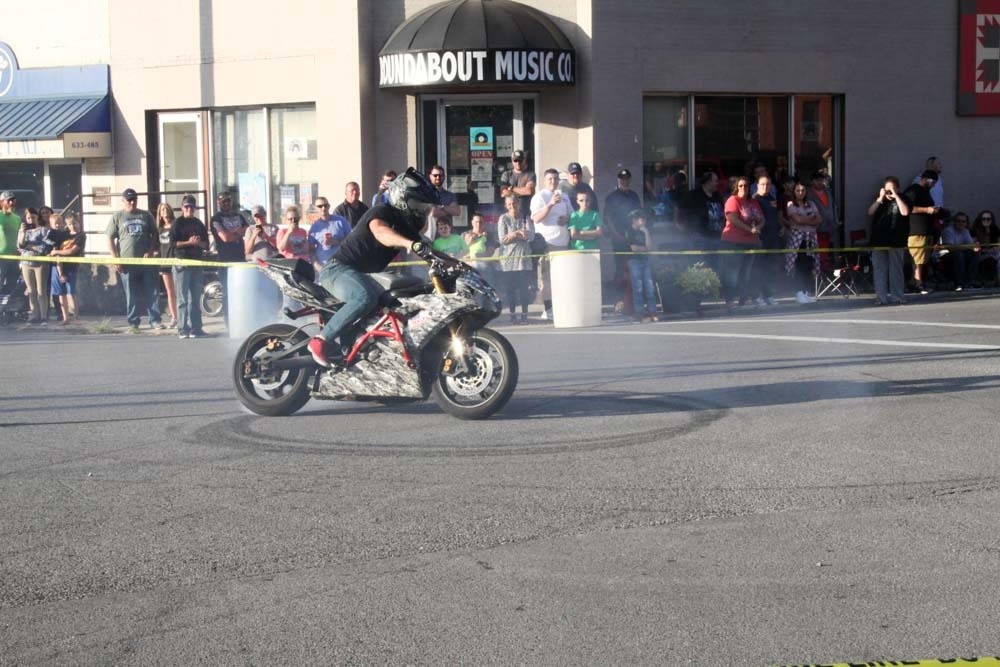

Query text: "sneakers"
[307, 338, 330, 368]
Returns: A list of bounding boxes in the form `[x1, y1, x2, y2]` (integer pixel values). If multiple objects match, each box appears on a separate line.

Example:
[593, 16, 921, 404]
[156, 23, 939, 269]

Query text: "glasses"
[431, 174, 442, 178]
[184, 205, 195, 209]
[514, 160, 522, 162]
[956, 220, 966, 223]
[316, 204, 328, 208]
[982, 217, 992, 221]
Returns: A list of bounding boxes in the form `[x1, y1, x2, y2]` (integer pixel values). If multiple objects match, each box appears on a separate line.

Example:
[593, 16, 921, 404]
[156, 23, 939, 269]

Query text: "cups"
[325, 233, 331, 245]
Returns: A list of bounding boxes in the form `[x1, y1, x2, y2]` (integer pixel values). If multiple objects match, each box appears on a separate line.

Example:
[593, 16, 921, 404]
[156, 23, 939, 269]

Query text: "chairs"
[813, 230, 862, 301]
[931, 217, 958, 291]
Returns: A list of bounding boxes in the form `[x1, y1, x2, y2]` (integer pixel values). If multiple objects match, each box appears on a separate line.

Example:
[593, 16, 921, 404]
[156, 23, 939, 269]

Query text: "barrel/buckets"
[227, 264, 307, 335]
[550, 249, 602, 327]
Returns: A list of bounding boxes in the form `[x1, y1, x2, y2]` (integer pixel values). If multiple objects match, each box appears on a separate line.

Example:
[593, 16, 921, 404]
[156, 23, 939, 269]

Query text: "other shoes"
[614, 275, 994, 324]
[125, 315, 211, 339]
[509, 310, 552, 325]
[26, 313, 78, 326]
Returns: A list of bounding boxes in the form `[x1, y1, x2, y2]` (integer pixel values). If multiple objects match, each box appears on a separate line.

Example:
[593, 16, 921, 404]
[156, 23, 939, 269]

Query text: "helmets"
[388, 167, 440, 235]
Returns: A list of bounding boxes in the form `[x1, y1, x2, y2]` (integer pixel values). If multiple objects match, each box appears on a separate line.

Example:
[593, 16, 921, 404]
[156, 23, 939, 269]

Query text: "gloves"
[407, 240, 432, 259]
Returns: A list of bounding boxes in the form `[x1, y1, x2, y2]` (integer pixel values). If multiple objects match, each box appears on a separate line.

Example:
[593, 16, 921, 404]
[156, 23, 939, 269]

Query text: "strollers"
[0, 267, 28, 326]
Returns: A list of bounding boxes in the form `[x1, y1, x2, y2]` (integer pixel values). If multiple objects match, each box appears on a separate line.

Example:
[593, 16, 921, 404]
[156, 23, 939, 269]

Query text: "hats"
[629, 209, 649, 218]
[251, 205, 264, 217]
[568, 163, 582, 174]
[123, 189, 137, 200]
[512, 150, 524, 161]
[918, 170, 938, 181]
[618, 169, 631, 179]
[217, 192, 232, 202]
[0, 191, 15, 200]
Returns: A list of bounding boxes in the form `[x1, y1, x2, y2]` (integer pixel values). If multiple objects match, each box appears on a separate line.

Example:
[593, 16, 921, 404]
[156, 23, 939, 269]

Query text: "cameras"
[884, 190, 892, 195]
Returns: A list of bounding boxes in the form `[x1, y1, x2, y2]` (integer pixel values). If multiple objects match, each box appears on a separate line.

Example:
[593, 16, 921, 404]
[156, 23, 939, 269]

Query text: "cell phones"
[255, 224, 263, 238]
[25, 224, 36, 229]
[554, 189, 562, 195]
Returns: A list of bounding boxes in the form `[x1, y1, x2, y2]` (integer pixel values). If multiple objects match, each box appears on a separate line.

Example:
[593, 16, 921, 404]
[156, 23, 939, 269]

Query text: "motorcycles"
[231, 241, 520, 421]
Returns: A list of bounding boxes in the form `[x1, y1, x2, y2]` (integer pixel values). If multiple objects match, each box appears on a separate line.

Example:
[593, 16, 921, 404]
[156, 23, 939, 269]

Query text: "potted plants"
[672, 265, 721, 308]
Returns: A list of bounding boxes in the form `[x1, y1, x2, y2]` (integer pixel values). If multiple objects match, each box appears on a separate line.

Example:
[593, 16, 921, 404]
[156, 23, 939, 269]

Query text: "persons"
[0, 191, 87, 326]
[904, 156, 1000, 294]
[867, 175, 913, 305]
[167, 195, 210, 339]
[106, 188, 167, 330]
[210, 149, 822, 331]
[155, 202, 177, 329]
[307, 166, 442, 369]
